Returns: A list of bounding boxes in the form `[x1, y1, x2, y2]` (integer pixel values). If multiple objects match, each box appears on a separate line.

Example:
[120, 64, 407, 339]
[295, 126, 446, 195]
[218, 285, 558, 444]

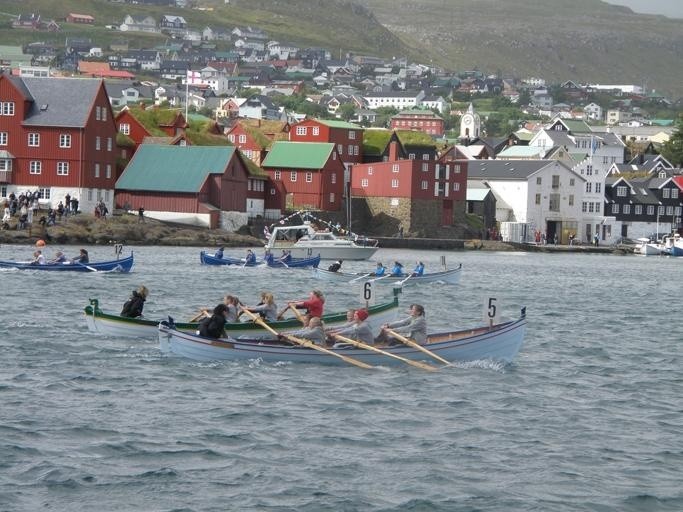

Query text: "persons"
[398, 222, 404, 239]
[194, 304, 230, 340]
[214, 245, 224, 259]
[477, 226, 599, 245]
[263, 225, 271, 239]
[119, 284, 148, 319]
[1, 188, 146, 265]
[200, 288, 428, 351]
[245, 248, 426, 278]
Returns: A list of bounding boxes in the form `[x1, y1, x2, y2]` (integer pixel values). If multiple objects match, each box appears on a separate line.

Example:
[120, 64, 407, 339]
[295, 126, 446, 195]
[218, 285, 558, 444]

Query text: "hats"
[354, 309, 368, 321]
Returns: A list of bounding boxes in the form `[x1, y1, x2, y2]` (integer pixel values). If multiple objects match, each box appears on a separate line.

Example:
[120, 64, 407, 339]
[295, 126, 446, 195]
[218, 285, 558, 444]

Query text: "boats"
[632, 228, 682, 256]
[157, 306, 526, 367]
[0, 250, 133, 273]
[313, 262, 462, 287]
[263, 207, 379, 263]
[200, 250, 319, 269]
[83, 287, 402, 340]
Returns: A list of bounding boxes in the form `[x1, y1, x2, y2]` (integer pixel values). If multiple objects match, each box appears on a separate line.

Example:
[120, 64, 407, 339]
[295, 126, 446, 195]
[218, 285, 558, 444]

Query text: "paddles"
[75, 260, 97, 272]
[288, 335, 373, 368]
[382, 328, 450, 364]
[331, 332, 438, 370]
